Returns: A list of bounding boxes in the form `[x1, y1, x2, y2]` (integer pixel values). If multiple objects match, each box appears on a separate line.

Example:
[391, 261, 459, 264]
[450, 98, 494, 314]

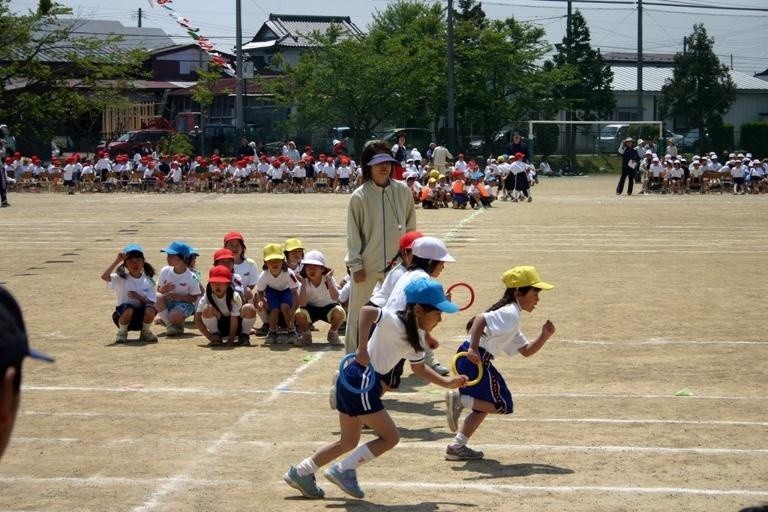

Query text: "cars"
[663, 130, 684, 146]
[469, 132, 529, 155]
[684, 129, 704, 146]
[51, 136, 74, 159]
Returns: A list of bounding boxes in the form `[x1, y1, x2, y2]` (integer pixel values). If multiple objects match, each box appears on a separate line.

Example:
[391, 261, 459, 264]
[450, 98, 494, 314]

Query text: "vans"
[0, 123, 17, 156]
[597, 124, 641, 151]
[377, 128, 432, 152]
[108, 130, 173, 155]
[329, 127, 377, 156]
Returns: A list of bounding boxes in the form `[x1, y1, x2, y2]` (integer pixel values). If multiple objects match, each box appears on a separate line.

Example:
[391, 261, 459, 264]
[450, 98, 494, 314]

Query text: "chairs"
[639, 155, 768, 195]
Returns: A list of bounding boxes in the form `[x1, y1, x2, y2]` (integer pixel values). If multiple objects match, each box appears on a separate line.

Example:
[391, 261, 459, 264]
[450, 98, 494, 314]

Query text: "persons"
[155, 240, 201, 338]
[277, 238, 320, 335]
[617, 137, 639, 196]
[0, 127, 12, 208]
[293, 251, 346, 347]
[4, 137, 361, 197]
[356, 229, 426, 352]
[281, 277, 469, 500]
[213, 248, 255, 335]
[154, 245, 205, 324]
[194, 265, 256, 346]
[257, 243, 299, 344]
[403, 131, 543, 209]
[634, 139, 768, 195]
[445, 264, 555, 462]
[253, 292, 270, 337]
[0, 283, 54, 463]
[101, 243, 158, 344]
[391, 135, 408, 161]
[344, 140, 450, 377]
[328, 236, 452, 429]
[336, 251, 353, 335]
[224, 231, 259, 290]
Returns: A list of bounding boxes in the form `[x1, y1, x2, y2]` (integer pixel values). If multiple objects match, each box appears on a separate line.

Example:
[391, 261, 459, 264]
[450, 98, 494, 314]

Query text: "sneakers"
[445, 444, 484, 461]
[283, 466, 323, 499]
[111, 326, 342, 346]
[324, 465, 364, 498]
[446, 392, 463, 431]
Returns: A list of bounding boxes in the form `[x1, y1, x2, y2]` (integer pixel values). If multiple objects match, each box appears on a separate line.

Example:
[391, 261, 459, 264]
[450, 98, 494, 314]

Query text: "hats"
[624, 138, 767, 168]
[3, 151, 352, 169]
[399, 231, 423, 252]
[513, 132, 520, 135]
[1, 287, 55, 362]
[369, 151, 399, 167]
[121, 231, 331, 284]
[501, 266, 555, 290]
[407, 148, 524, 185]
[344, 253, 349, 266]
[404, 278, 459, 313]
[411, 236, 456, 262]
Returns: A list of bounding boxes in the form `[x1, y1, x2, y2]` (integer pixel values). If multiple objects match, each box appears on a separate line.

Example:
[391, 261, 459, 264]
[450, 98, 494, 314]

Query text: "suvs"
[189, 124, 236, 138]
